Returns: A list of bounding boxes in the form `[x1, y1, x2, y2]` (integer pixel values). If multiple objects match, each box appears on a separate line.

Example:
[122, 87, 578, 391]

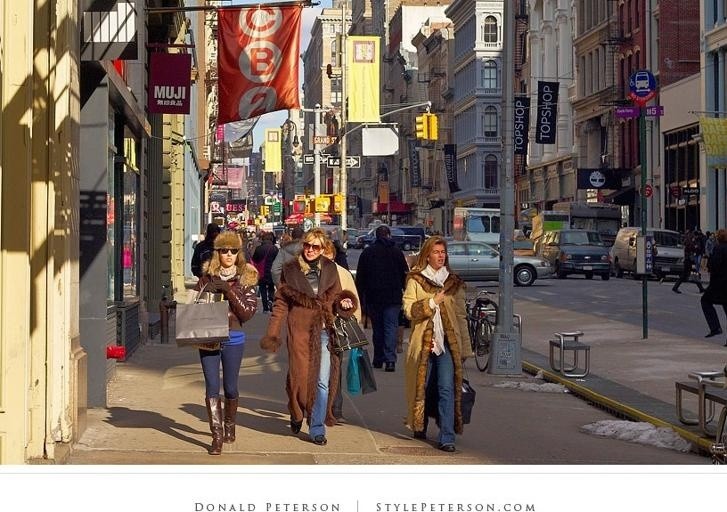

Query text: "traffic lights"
[334, 196, 343, 210]
[414, 113, 428, 141]
[293, 200, 306, 213]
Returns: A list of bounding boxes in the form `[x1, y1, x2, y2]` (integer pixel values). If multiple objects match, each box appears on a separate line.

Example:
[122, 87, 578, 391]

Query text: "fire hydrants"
[158, 292, 177, 345]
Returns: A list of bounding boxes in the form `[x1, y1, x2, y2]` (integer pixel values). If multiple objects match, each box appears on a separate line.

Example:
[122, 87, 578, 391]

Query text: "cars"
[271, 211, 427, 252]
[440, 237, 554, 287]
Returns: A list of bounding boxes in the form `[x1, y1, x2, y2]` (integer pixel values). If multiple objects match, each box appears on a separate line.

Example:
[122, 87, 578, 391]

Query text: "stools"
[549, 329, 590, 377]
[468, 308, 522, 348]
[677, 366, 727, 438]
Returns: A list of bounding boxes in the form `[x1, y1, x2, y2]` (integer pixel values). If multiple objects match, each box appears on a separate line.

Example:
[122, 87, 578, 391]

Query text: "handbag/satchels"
[329, 312, 370, 356]
[175, 282, 231, 352]
[700, 255, 709, 273]
[460, 360, 476, 423]
[249, 256, 266, 281]
[398, 303, 411, 328]
[346, 346, 378, 396]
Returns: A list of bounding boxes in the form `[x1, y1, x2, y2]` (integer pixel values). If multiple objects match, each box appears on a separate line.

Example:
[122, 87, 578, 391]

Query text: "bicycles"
[464, 289, 497, 372]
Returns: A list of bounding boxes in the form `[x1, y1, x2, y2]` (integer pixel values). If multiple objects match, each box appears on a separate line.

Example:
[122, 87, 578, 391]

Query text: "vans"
[513, 201, 688, 285]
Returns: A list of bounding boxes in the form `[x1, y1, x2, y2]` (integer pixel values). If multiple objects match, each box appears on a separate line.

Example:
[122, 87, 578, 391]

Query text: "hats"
[207, 223, 222, 233]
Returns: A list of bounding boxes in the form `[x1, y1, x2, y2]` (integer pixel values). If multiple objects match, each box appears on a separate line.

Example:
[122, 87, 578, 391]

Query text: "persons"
[254, 228, 358, 446]
[187, 232, 260, 455]
[699, 230, 726, 348]
[188, 219, 355, 316]
[357, 224, 413, 374]
[395, 237, 478, 452]
[670, 226, 726, 298]
[396, 302, 412, 353]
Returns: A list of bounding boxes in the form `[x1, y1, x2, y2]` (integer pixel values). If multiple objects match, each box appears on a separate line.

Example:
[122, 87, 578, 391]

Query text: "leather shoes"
[704, 327, 723, 338]
[312, 434, 327, 445]
[438, 441, 455, 452]
[414, 416, 428, 439]
[290, 408, 304, 434]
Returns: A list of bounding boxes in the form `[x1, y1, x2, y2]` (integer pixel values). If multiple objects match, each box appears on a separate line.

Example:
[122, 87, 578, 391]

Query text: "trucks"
[451, 205, 502, 248]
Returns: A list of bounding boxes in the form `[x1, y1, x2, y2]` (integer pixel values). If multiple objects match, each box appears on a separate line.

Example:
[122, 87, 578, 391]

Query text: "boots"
[205, 396, 223, 455]
[397, 325, 405, 353]
[223, 397, 238, 443]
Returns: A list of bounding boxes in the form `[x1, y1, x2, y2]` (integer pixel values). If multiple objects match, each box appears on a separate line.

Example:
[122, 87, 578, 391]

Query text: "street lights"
[280, 118, 300, 224]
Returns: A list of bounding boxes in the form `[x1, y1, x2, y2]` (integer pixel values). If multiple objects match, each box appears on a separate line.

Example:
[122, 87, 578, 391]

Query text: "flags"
[343, 34, 382, 123]
[214, 4, 305, 127]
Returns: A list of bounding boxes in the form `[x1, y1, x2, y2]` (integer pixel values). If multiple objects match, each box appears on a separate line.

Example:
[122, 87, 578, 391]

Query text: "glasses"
[215, 248, 240, 255]
[302, 241, 324, 253]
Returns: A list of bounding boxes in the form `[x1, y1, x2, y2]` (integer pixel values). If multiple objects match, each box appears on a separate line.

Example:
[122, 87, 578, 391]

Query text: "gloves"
[206, 276, 232, 293]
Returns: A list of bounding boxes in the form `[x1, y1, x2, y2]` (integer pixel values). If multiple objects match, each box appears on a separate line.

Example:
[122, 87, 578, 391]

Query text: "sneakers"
[372, 359, 383, 368]
[267, 300, 273, 312]
[336, 417, 352, 423]
[385, 360, 396, 372]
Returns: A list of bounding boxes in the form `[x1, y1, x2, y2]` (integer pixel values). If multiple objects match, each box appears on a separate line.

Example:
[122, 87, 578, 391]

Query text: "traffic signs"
[326, 155, 361, 169]
[312, 135, 339, 144]
[304, 153, 332, 165]
